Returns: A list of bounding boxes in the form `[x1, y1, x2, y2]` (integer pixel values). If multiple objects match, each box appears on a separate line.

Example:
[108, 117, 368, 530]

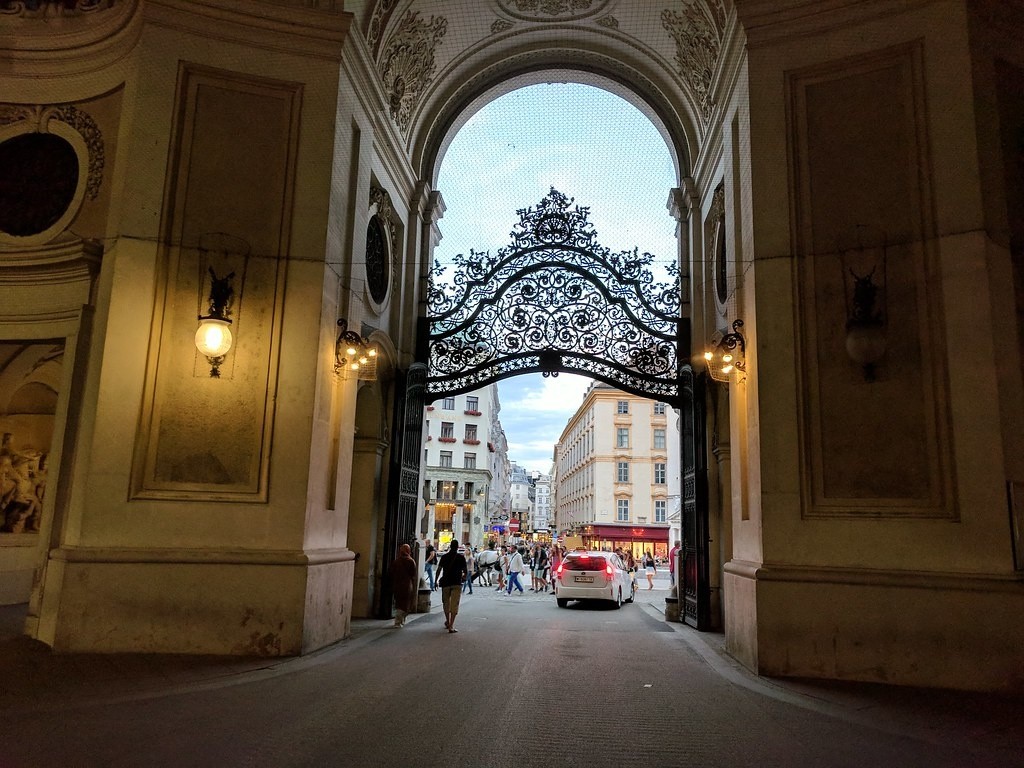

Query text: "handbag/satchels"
[543, 559, 551, 569]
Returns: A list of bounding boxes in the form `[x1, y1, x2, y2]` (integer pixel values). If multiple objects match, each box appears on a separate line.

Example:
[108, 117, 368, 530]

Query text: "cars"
[555, 546, 635, 610]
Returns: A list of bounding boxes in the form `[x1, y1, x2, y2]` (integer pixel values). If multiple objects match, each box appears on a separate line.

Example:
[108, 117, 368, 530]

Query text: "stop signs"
[509, 518, 519, 532]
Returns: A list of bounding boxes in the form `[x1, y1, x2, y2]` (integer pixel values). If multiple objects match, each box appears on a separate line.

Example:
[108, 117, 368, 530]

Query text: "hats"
[399, 544, 411, 558]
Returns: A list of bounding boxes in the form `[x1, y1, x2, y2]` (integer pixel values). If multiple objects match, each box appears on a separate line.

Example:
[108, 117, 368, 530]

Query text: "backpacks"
[494, 557, 506, 571]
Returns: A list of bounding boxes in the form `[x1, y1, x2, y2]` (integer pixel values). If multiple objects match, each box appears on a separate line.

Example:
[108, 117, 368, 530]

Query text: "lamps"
[194, 267, 236, 378]
[839, 264, 887, 383]
[703, 320, 746, 374]
[332, 317, 378, 375]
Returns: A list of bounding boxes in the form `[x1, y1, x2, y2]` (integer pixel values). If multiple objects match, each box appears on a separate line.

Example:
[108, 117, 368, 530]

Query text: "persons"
[488, 535, 569, 597]
[669, 540, 681, 588]
[391, 544, 417, 628]
[0, 433, 51, 534]
[584, 542, 669, 593]
[435, 540, 468, 634]
[424, 537, 478, 595]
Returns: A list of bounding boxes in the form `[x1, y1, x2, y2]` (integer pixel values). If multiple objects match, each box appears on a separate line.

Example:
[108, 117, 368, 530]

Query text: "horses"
[474, 550, 500, 587]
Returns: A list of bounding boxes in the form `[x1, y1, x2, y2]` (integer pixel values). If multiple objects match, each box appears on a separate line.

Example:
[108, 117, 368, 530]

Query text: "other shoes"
[467, 592, 472, 594]
[539, 587, 543, 591]
[495, 588, 502, 591]
[498, 584, 505, 588]
[545, 585, 549, 592]
[528, 588, 534, 590]
[445, 621, 449, 628]
[649, 585, 654, 589]
[449, 629, 458, 633]
[395, 623, 403, 627]
[517, 589, 522, 596]
[533, 590, 539, 594]
[504, 593, 511, 597]
[550, 591, 555, 595]
